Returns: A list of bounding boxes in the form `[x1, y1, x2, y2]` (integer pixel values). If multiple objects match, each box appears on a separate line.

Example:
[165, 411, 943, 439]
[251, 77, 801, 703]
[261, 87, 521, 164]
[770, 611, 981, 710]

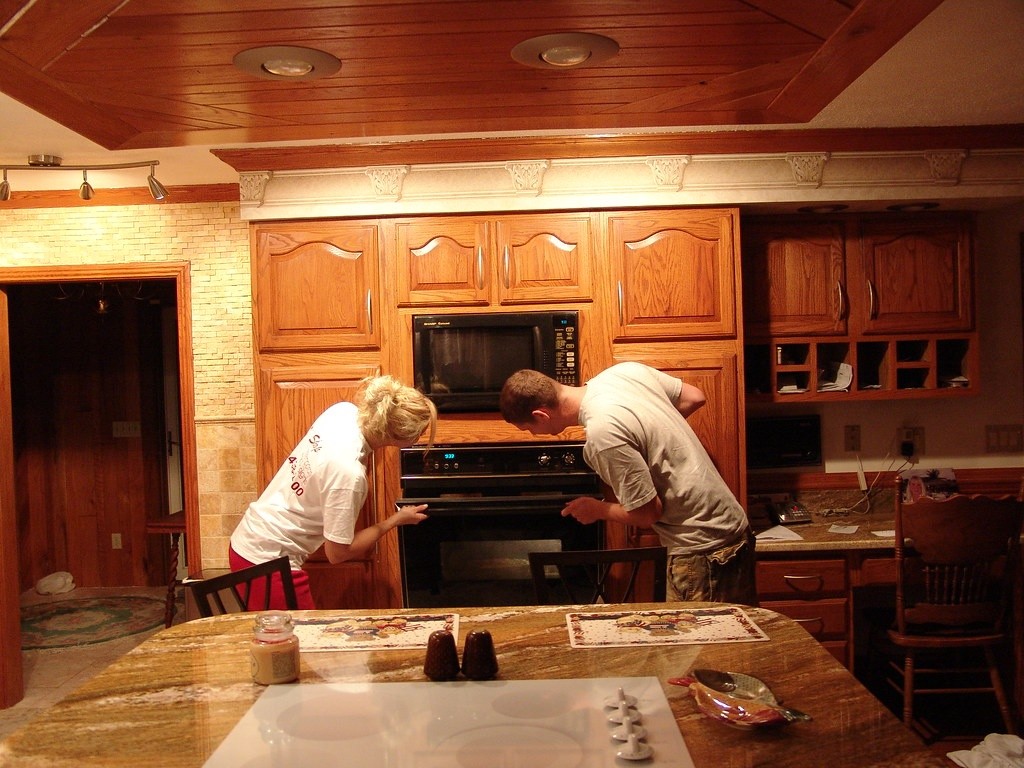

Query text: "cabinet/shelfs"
[743, 211, 982, 400]
[249, 204, 746, 610]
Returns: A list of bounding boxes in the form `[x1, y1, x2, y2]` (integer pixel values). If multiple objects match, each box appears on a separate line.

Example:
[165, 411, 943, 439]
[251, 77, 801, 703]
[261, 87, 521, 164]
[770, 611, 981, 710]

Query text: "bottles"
[249, 610, 300, 685]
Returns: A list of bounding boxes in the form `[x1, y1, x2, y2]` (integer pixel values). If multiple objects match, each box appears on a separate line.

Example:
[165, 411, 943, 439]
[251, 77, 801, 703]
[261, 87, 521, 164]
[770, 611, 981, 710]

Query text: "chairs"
[190, 554, 298, 619]
[886, 476, 1024, 738]
[528, 547, 667, 604]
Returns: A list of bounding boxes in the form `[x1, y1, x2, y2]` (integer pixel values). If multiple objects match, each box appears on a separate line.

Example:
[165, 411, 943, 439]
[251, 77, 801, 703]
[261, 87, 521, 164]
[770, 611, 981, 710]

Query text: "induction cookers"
[197, 675, 695, 768]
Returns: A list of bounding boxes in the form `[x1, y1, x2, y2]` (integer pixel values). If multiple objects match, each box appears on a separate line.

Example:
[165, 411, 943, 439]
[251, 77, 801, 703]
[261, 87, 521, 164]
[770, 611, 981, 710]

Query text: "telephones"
[768, 499, 812, 523]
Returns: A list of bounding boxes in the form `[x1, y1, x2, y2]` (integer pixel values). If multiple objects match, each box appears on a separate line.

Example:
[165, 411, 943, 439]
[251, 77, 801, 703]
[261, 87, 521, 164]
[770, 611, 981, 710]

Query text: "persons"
[226, 373, 435, 609]
[500, 361, 756, 603]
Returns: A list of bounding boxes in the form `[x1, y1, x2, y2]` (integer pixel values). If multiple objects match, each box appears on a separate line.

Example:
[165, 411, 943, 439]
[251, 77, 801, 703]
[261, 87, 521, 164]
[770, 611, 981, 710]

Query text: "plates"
[668, 668, 814, 730]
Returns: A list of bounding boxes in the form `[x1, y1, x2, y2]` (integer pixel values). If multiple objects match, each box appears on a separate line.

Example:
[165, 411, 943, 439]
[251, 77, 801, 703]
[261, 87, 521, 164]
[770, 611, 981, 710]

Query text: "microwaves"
[410, 310, 579, 415]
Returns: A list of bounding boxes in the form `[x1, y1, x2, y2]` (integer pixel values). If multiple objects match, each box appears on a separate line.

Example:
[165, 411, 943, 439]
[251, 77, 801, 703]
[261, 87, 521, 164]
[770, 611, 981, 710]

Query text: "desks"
[746, 487, 922, 676]
[147, 509, 185, 628]
[1, 601, 959, 768]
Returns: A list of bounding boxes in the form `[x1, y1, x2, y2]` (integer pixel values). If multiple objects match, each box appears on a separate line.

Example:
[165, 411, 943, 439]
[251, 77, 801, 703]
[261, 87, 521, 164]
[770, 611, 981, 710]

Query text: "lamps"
[44, 281, 163, 313]
[0, 155, 169, 200]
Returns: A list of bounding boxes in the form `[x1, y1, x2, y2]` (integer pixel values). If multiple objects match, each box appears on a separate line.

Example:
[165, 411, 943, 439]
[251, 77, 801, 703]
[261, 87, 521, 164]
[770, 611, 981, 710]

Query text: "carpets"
[20, 595, 178, 651]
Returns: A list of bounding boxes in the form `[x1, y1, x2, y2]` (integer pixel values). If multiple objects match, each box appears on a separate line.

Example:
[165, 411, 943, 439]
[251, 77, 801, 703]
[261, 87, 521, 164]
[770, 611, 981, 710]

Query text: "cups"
[461, 629, 498, 681]
[424, 630, 460, 681]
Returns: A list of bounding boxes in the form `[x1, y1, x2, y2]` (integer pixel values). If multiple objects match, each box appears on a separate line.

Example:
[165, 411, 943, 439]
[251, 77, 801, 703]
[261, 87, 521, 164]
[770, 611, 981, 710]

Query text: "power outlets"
[845, 426, 860, 451]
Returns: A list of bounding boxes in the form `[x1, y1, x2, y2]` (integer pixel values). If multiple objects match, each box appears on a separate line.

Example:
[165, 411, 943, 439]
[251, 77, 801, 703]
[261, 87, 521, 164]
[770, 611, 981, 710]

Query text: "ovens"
[394, 447, 607, 607]
